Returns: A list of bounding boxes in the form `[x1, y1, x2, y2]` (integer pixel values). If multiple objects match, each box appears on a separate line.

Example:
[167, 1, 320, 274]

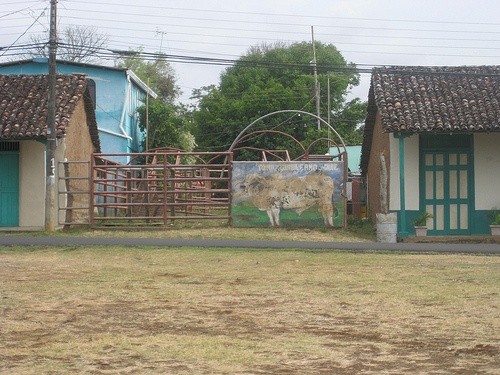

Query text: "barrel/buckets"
[375, 212, 398, 244]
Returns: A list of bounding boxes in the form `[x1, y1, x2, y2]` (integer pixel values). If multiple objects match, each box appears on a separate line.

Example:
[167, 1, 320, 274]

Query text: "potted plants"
[487, 208, 500, 236]
[414, 212, 435, 236]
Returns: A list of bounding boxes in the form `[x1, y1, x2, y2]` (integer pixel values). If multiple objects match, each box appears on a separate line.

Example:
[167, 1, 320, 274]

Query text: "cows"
[239, 172, 334, 226]
